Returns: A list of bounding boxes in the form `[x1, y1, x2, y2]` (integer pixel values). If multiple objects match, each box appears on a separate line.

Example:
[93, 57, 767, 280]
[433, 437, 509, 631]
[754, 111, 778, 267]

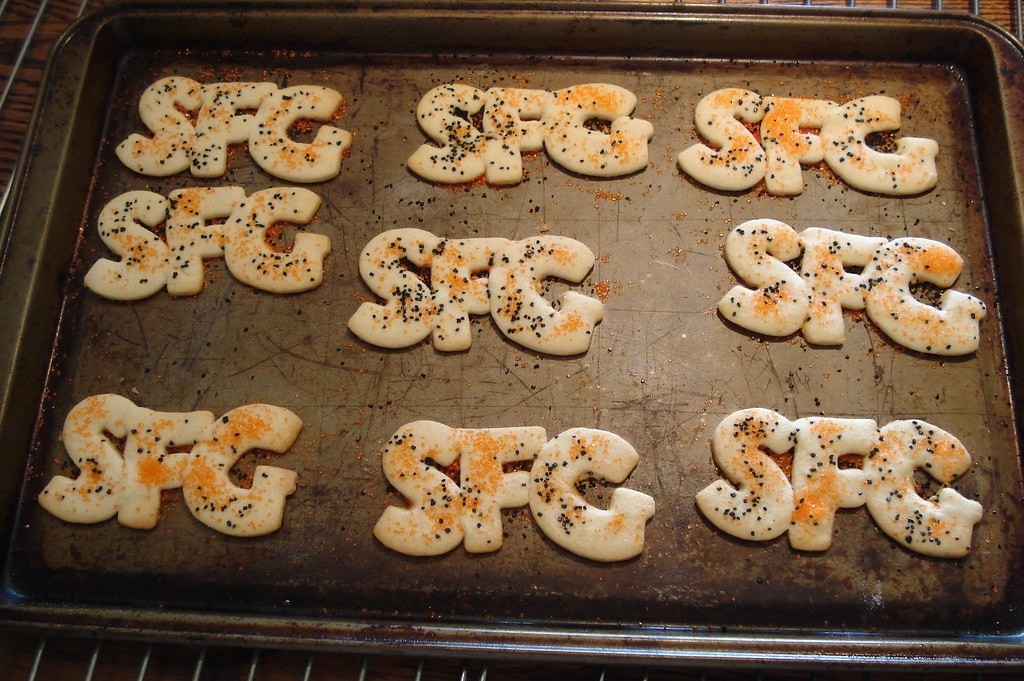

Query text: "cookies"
[39, 77, 987, 557]
